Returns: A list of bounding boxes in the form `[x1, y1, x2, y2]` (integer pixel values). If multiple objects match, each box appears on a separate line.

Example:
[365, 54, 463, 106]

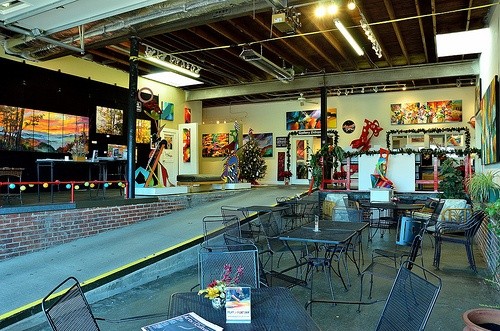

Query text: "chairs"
[41, 197, 487, 331]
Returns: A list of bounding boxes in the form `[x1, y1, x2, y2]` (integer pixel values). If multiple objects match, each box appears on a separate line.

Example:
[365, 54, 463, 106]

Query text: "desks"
[361, 202, 424, 241]
[94, 157, 127, 201]
[238, 206, 289, 254]
[37, 158, 99, 203]
[279, 229, 356, 300]
[168, 287, 321, 331]
[0, 168, 25, 205]
[276, 200, 319, 231]
[302, 221, 368, 282]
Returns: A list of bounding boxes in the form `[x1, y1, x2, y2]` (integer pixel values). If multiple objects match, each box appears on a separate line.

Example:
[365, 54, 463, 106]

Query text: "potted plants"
[67, 137, 89, 161]
[465, 170, 500, 203]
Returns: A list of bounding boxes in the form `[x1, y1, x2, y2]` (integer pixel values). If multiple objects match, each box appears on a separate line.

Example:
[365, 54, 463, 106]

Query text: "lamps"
[334, 84, 406, 96]
[239, 45, 295, 84]
[140, 42, 201, 78]
[315, 0, 383, 58]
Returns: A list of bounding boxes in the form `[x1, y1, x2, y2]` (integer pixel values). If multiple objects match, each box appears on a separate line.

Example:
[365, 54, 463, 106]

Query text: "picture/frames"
[276, 137, 287, 147]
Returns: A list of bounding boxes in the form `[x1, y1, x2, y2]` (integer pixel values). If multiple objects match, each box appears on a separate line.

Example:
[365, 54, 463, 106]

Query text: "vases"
[211, 298, 226, 311]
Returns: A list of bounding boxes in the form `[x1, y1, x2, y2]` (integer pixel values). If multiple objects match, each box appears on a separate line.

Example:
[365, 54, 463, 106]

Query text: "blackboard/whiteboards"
[370, 191, 390, 227]
[358, 153, 416, 191]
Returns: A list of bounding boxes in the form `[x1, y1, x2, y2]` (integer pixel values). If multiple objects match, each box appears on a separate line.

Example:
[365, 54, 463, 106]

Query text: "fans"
[297, 93, 318, 106]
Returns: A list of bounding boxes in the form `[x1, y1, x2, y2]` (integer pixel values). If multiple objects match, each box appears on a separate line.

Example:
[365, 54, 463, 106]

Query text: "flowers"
[197, 264, 254, 300]
